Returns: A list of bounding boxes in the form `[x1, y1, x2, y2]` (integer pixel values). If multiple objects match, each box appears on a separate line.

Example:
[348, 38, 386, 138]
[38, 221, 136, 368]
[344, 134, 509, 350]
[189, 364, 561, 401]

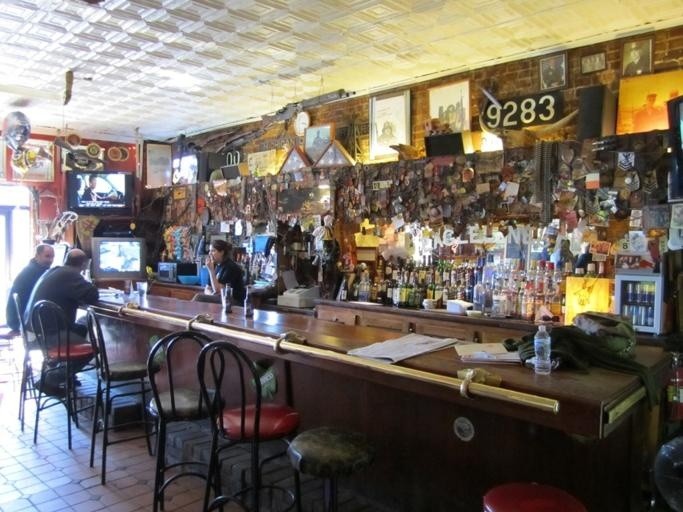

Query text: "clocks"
[293, 111, 310, 137]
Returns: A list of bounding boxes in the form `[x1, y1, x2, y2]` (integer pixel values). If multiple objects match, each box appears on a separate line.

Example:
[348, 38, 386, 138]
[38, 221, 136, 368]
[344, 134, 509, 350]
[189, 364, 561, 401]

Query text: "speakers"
[577, 85, 616, 141]
[424, 133, 473, 158]
[197, 151, 227, 183]
[221, 165, 242, 181]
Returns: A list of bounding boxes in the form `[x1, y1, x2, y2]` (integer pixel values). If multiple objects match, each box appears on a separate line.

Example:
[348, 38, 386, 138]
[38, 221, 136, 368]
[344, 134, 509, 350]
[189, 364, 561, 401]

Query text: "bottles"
[222, 283, 233, 313]
[338, 253, 608, 321]
[242, 286, 254, 318]
[533, 325, 552, 375]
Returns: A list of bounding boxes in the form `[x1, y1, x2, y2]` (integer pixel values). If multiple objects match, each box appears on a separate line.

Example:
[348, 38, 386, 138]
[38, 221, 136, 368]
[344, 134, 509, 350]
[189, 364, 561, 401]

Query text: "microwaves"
[157, 262, 196, 283]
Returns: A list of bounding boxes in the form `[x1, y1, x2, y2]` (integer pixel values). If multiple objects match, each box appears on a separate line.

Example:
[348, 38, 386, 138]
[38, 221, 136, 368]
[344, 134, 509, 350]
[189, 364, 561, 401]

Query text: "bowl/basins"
[177, 275, 200, 285]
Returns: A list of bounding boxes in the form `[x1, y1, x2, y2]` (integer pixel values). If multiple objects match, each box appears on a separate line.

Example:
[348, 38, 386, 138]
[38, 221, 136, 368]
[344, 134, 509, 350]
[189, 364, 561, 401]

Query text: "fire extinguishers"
[666, 351, 683, 422]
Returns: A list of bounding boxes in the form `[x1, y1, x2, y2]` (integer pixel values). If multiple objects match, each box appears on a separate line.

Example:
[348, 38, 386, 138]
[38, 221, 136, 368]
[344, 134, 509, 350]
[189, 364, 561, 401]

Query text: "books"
[453, 342, 522, 365]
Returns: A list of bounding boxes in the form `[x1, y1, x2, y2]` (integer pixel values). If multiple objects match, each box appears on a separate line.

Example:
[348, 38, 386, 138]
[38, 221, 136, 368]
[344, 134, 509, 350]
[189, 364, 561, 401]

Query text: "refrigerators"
[614, 273, 674, 335]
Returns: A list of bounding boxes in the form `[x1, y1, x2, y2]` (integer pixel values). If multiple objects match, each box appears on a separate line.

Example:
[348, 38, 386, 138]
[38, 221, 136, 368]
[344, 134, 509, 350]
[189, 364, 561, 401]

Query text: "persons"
[82, 175, 118, 201]
[22, 247, 98, 397]
[191, 239, 244, 306]
[4, 244, 54, 333]
[75, 174, 83, 207]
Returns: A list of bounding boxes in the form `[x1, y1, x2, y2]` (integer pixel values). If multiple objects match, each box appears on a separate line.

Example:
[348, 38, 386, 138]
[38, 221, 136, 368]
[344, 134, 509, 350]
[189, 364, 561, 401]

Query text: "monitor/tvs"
[172, 155, 198, 184]
[67, 169, 135, 216]
[667, 96, 683, 167]
[91, 236, 147, 282]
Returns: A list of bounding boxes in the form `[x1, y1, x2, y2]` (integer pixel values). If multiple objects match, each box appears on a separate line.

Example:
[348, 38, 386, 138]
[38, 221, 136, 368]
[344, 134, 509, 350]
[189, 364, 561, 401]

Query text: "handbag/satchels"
[571, 308, 639, 357]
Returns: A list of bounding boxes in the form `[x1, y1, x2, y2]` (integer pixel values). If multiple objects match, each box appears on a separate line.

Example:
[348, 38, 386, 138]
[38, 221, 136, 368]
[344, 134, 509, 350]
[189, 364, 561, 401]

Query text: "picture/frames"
[536, 52, 569, 92]
[620, 35, 654, 77]
[369, 89, 413, 161]
[301, 121, 336, 164]
[425, 76, 473, 133]
[10, 134, 56, 184]
[614, 66, 683, 135]
[579, 50, 607, 74]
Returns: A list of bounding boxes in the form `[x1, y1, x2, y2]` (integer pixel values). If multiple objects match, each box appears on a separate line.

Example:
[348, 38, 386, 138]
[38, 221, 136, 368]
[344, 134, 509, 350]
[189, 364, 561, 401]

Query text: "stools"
[290, 422, 387, 511]
[145, 328, 225, 511]
[655, 432, 683, 512]
[482, 480, 590, 512]
[28, 299, 97, 449]
[195, 337, 300, 509]
[85, 303, 155, 488]
[10, 293, 80, 431]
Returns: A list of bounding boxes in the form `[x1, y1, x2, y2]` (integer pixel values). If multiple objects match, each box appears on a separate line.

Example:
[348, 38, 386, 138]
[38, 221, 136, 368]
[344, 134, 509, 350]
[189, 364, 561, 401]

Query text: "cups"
[135, 282, 147, 298]
